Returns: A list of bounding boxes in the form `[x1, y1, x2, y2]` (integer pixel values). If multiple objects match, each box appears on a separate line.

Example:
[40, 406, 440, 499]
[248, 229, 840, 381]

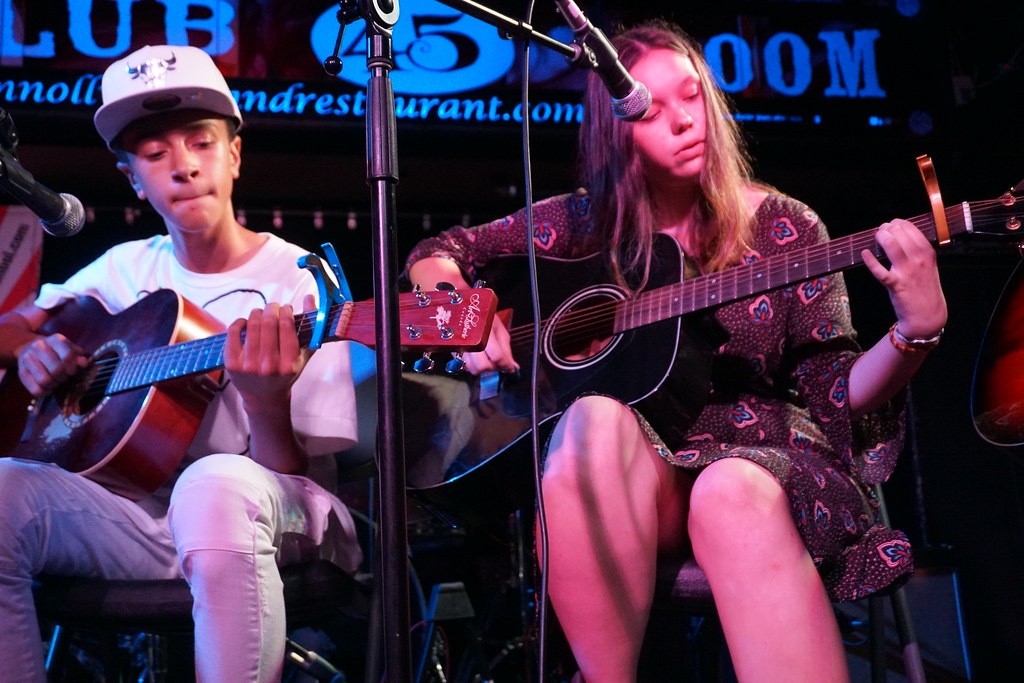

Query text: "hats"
[93, 44, 243, 153]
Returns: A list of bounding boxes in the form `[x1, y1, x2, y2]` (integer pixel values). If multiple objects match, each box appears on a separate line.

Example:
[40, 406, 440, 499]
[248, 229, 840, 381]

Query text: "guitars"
[966, 249, 1023, 448]
[371, 180, 1024, 507]
[0, 278, 500, 502]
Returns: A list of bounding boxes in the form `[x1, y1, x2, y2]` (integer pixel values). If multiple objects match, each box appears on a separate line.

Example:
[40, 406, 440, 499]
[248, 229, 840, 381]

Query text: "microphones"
[0, 146, 84, 235]
[554, 0, 652, 121]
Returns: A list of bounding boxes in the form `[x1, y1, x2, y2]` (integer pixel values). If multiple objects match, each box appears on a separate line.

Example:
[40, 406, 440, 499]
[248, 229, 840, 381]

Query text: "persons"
[0, 44, 358, 682]
[406, 28, 948, 683]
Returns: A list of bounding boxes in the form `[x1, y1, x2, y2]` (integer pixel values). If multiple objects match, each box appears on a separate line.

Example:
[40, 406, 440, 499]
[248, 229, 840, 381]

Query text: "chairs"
[514, 452, 924, 683]
[33, 372, 443, 683]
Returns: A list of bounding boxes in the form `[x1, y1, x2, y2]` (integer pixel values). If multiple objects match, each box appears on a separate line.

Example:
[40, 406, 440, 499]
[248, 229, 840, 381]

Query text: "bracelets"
[895, 325, 944, 347]
[889, 321, 939, 354]
